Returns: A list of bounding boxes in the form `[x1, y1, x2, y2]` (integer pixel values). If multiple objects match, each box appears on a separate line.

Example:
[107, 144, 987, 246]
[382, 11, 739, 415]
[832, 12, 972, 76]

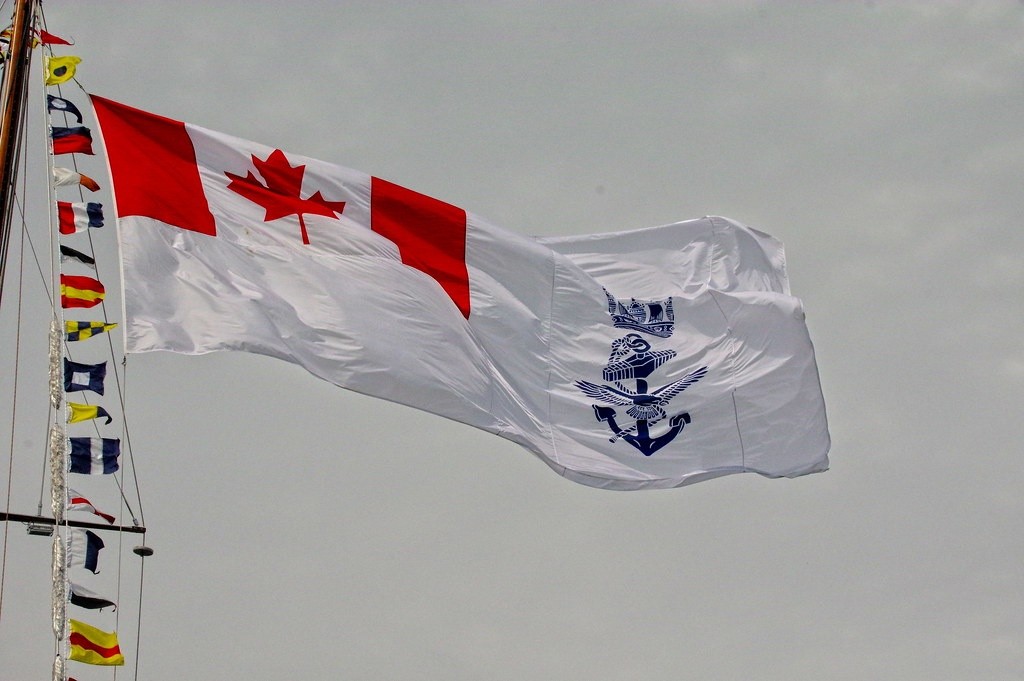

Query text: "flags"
[91, 95, 831, 489]
[39, 31, 127, 668]
